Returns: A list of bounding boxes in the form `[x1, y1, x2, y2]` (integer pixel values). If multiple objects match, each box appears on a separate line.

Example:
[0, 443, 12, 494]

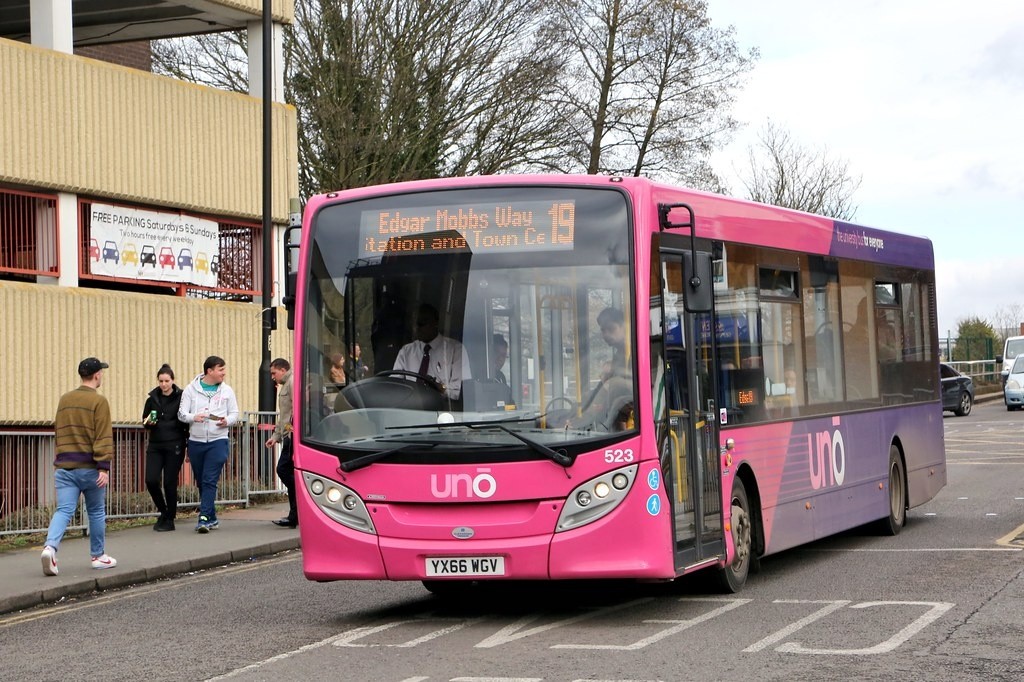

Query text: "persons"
[387, 306, 471, 415]
[542, 307, 658, 434]
[265, 358, 299, 528]
[142, 364, 190, 530]
[331, 342, 369, 384]
[177, 356, 238, 531]
[475, 334, 516, 412]
[40, 357, 117, 575]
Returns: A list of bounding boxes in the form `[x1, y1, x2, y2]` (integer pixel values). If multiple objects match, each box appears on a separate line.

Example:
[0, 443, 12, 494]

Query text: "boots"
[154, 512, 175, 531]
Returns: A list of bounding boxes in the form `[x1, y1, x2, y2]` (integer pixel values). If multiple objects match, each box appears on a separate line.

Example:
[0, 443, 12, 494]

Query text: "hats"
[331, 353, 343, 364]
[79, 358, 108, 372]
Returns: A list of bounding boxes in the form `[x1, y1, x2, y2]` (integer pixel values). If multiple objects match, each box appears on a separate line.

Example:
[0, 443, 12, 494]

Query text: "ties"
[415, 344, 432, 382]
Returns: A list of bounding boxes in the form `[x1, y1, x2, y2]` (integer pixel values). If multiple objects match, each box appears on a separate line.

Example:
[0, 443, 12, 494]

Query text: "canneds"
[150, 410, 157, 423]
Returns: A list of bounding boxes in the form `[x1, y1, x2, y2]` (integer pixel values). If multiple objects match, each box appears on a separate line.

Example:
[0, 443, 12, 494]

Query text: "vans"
[996, 335, 1024, 404]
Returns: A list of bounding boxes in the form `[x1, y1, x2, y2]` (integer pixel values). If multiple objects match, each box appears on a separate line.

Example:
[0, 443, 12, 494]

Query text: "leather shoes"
[271, 517, 298, 529]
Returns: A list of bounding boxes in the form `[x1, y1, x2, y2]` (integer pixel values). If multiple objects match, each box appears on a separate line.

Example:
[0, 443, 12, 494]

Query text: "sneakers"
[91, 553, 116, 569]
[195, 515, 218, 533]
[41, 547, 58, 575]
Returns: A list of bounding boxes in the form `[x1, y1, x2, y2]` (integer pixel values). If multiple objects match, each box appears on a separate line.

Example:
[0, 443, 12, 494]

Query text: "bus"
[282, 176, 950, 596]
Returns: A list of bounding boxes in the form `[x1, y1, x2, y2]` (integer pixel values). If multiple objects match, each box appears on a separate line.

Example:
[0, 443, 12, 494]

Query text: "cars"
[1000, 354, 1024, 411]
[939, 363, 974, 417]
